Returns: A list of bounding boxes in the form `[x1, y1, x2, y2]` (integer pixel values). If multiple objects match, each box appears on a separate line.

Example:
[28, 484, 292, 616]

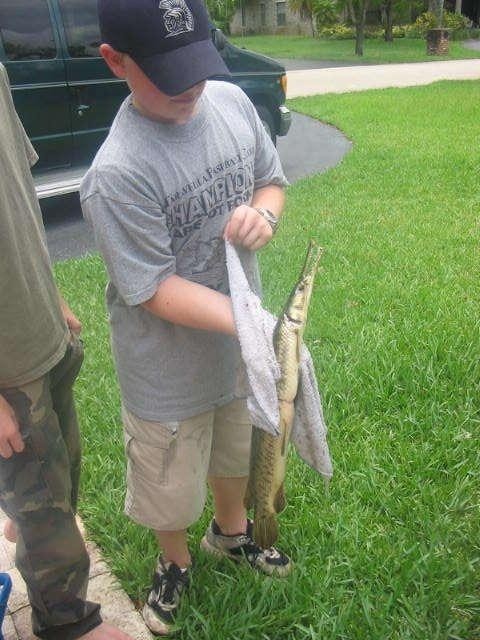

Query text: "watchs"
[254, 206, 278, 233]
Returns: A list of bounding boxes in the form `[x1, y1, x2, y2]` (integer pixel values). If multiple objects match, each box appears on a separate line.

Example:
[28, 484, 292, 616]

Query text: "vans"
[0, 0, 292, 205]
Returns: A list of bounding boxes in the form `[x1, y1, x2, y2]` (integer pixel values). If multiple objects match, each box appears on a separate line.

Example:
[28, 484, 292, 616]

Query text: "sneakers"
[198, 515, 298, 579]
[142, 551, 194, 636]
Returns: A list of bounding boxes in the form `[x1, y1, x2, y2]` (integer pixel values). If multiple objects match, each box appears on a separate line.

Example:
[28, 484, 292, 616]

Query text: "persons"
[80, 1, 296, 637]
[0, 62, 134, 640]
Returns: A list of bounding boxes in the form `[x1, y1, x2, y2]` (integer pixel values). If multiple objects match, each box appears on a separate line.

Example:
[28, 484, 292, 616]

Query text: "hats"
[97, 0, 233, 98]
